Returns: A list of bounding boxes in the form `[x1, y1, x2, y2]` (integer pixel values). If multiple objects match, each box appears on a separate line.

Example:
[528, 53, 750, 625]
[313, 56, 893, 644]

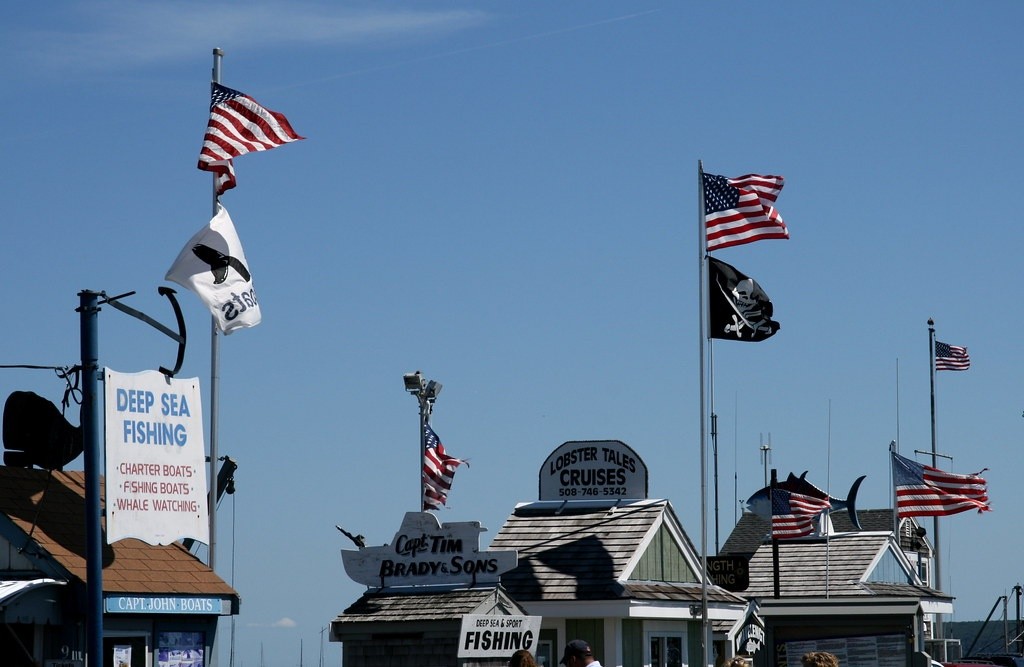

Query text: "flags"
[709, 256, 781, 342]
[198, 83, 307, 200]
[771, 487, 832, 539]
[935, 340, 970, 370]
[894, 451, 992, 517]
[165, 200, 261, 335]
[702, 172, 789, 251]
[423, 421, 470, 512]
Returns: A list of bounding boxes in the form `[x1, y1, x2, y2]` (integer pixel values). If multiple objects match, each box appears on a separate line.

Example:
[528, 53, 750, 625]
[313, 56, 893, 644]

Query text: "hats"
[560, 639, 591, 664]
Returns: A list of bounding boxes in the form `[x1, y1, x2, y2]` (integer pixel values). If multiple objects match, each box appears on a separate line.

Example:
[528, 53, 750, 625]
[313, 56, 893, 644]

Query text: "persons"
[801, 651, 839, 667]
[508, 650, 538, 667]
[559, 639, 603, 667]
